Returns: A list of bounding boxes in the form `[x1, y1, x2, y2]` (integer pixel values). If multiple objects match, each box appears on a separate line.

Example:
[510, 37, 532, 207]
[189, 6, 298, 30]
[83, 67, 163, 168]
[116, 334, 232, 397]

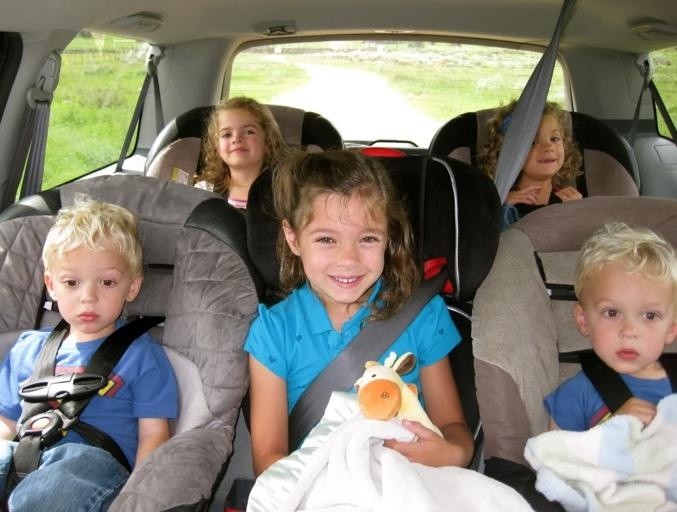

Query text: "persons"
[478, 97, 583, 220]
[541, 217, 676, 433]
[190, 95, 293, 209]
[1, 190, 181, 508]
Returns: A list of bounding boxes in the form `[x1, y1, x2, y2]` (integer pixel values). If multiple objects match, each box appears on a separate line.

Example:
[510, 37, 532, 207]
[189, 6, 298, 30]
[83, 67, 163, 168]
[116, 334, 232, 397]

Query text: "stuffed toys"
[351, 350, 443, 442]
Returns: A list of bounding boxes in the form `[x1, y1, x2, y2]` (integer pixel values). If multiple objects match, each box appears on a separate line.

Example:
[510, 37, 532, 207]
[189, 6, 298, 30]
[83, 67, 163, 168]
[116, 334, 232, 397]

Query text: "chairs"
[427, 111, 635, 196]
[471, 194, 677, 468]
[144, 103, 343, 188]
[0, 174, 266, 512]
